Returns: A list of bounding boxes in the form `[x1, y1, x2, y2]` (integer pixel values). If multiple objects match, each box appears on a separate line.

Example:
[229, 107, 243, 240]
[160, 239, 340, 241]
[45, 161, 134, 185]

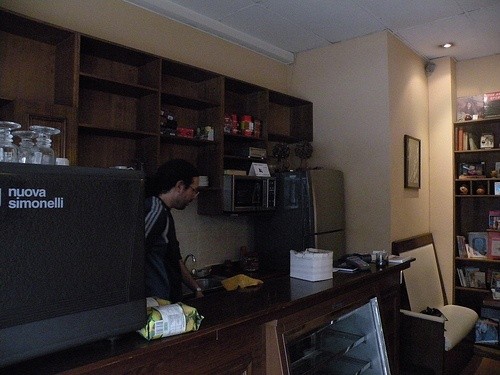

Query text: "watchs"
[193, 286, 202, 293]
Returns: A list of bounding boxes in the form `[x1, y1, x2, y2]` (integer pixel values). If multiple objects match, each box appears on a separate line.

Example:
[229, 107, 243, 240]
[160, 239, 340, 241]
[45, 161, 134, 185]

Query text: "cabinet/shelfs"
[453, 116, 500, 360]
[0, 7, 312, 216]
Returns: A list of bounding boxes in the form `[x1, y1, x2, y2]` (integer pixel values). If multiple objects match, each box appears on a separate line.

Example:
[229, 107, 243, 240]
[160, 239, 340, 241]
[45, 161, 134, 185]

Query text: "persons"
[141, 158, 205, 303]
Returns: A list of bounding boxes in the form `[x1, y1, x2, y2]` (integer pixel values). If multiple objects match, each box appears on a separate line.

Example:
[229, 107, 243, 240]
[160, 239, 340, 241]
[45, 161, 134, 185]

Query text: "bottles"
[476, 186, 485, 195]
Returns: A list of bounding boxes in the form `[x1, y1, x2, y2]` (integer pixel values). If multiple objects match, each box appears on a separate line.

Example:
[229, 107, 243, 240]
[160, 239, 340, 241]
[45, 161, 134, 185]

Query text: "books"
[455, 126, 479, 151]
[456, 231, 500, 289]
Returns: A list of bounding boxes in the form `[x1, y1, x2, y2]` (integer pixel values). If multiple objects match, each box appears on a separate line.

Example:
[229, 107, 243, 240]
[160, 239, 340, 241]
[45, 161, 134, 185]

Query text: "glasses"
[183, 181, 200, 200]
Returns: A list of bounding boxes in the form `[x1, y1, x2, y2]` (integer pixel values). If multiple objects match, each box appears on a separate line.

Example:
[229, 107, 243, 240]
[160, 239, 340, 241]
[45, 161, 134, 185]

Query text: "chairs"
[392, 232, 479, 375]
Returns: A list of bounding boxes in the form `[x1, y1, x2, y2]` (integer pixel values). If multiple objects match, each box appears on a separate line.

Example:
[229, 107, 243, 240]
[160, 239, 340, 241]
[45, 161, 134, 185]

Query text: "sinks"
[195, 279, 223, 290]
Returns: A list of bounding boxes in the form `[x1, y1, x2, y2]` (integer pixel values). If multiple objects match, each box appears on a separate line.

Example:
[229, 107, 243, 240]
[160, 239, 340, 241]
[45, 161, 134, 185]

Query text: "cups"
[376, 252, 388, 271]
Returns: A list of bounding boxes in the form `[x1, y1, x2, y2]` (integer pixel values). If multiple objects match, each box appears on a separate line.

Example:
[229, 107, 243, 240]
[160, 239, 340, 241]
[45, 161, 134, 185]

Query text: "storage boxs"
[469, 210, 500, 259]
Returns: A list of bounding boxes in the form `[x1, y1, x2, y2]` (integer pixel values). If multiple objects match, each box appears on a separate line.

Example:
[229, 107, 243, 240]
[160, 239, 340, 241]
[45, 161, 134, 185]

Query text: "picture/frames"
[403, 134, 421, 189]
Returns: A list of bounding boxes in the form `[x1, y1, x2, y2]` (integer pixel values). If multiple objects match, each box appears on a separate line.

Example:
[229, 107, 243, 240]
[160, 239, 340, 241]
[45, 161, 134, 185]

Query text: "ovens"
[196, 174, 277, 215]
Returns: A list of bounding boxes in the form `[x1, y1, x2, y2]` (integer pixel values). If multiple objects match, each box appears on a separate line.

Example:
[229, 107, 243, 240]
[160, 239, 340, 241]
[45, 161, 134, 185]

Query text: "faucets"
[183, 254, 196, 266]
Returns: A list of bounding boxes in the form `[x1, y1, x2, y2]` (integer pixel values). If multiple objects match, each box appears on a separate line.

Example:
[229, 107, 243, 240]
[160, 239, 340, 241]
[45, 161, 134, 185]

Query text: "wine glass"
[11, 129, 35, 163]
[28, 125, 61, 166]
[0, 120, 22, 162]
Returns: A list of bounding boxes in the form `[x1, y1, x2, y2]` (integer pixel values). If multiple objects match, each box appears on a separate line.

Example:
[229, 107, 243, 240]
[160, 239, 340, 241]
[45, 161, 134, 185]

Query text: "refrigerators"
[254, 168, 345, 276]
